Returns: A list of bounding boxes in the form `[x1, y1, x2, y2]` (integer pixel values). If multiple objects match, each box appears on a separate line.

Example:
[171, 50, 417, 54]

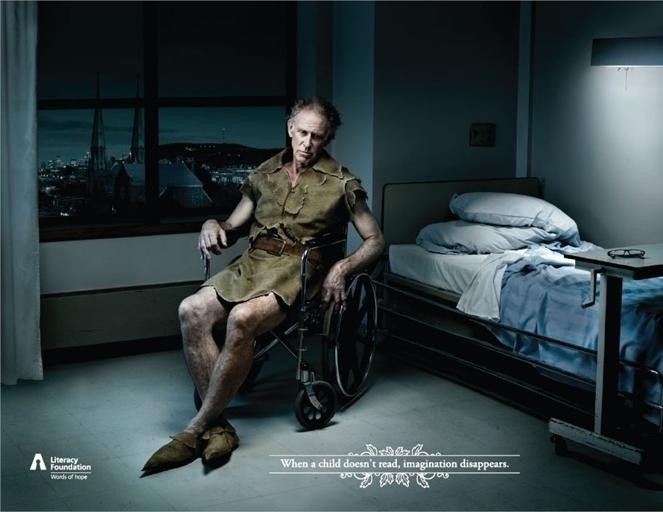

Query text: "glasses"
[607, 249, 645, 258]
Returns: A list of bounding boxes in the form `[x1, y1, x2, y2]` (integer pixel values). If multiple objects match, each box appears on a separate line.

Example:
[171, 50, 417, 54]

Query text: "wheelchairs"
[193, 213, 390, 432]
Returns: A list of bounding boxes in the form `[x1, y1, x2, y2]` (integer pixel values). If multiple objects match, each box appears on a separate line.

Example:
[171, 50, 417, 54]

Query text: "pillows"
[448, 191, 582, 250]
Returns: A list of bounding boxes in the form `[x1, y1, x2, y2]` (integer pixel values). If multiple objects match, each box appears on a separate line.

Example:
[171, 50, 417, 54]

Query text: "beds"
[375, 176, 663, 475]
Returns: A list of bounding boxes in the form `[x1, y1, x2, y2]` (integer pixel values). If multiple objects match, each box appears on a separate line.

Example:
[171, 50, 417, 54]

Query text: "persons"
[139, 95, 386, 476]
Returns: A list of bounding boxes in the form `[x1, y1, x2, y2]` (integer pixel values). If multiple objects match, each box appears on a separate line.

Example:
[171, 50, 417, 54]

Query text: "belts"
[252, 240, 322, 263]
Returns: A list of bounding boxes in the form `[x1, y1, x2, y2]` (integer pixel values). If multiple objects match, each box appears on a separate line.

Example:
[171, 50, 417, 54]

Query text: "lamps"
[590, 36, 663, 89]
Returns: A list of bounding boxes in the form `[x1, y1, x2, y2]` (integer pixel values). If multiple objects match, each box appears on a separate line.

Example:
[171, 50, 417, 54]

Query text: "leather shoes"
[141, 434, 204, 469]
[204, 426, 237, 465]
